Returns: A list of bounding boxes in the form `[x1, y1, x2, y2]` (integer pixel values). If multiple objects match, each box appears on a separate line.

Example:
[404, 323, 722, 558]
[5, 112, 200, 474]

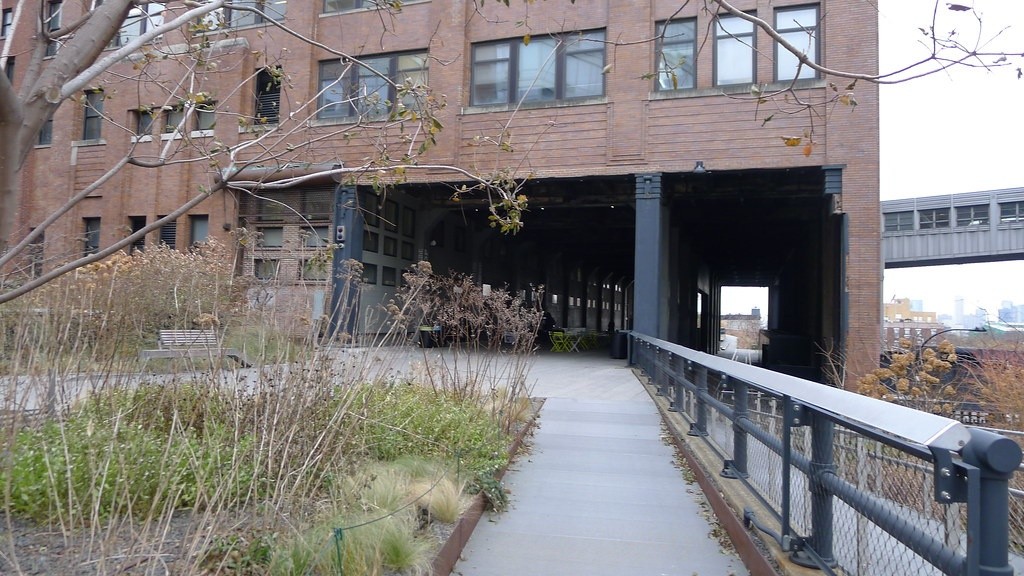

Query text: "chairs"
[547, 327, 603, 352]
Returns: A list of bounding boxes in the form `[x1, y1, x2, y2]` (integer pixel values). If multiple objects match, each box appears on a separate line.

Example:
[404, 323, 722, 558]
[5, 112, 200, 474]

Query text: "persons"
[607, 319, 616, 332]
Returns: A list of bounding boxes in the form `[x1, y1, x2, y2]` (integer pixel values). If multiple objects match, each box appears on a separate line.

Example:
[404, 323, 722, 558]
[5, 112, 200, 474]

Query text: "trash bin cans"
[418, 324, 442, 348]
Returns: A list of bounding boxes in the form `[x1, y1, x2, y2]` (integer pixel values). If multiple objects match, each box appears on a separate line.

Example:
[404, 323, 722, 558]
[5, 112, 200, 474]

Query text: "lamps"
[692, 160, 706, 173]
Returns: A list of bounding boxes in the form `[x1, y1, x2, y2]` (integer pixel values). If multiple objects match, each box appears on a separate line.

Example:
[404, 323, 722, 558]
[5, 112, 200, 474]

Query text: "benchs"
[139, 329, 252, 372]
